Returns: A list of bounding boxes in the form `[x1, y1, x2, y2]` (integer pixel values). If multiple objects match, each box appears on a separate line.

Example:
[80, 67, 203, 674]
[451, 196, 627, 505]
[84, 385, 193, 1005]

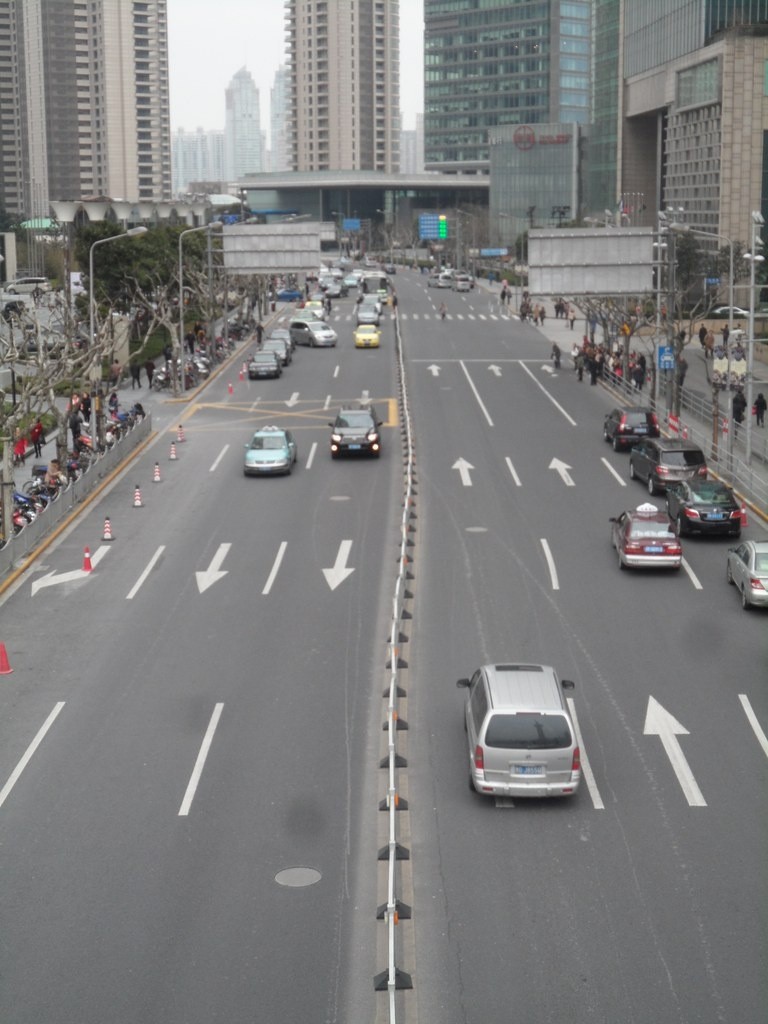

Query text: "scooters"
[0, 404, 143, 553]
[151, 316, 254, 394]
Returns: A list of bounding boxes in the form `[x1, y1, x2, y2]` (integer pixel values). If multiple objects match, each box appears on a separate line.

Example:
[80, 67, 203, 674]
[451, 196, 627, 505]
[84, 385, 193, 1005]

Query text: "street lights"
[90, 227, 148, 348]
[179, 221, 221, 391]
[666, 223, 732, 477]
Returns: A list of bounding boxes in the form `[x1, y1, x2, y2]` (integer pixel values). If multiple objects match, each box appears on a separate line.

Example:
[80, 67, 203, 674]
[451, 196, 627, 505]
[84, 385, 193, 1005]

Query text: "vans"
[427, 269, 474, 292]
[5, 277, 52, 295]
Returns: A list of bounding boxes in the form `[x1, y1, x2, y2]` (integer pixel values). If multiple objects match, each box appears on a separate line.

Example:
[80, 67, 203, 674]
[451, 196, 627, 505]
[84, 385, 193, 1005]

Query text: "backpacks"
[70, 414, 78, 429]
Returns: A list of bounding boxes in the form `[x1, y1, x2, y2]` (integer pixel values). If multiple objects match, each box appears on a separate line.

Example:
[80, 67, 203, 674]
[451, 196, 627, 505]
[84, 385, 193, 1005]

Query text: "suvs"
[457, 663, 581, 798]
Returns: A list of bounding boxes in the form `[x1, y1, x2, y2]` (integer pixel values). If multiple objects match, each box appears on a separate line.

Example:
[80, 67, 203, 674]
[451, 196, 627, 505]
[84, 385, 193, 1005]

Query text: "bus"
[359, 271, 390, 304]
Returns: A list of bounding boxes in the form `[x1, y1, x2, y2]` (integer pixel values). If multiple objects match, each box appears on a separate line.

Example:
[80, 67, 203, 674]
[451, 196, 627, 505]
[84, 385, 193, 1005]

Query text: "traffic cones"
[176, 424, 186, 442]
[168, 442, 179, 462]
[132, 485, 144, 508]
[150, 462, 163, 483]
[100, 517, 115, 541]
[81, 546, 94, 571]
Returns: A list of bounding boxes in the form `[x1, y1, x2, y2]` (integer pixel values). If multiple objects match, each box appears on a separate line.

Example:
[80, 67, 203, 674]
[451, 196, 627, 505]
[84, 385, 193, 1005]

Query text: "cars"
[247, 296, 382, 380]
[712, 306, 749, 316]
[243, 407, 383, 477]
[603, 406, 768, 610]
[304, 264, 361, 298]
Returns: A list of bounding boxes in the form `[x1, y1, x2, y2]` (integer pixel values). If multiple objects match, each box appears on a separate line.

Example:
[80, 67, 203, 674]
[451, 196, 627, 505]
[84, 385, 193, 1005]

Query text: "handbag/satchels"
[636, 363, 641, 369]
[36, 428, 46, 444]
[752, 405, 757, 414]
[631, 378, 636, 386]
[109, 405, 117, 412]
[740, 415, 744, 420]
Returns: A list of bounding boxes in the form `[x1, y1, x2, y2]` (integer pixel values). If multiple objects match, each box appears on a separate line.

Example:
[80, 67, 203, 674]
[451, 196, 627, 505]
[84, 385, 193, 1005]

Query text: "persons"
[520, 290, 545, 326]
[488, 271, 512, 305]
[254, 323, 265, 344]
[184, 320, 208, 354]
[551, 342, 562, 369]
[29, 416, 43, 459]
[554, 300, 576, 330]
[144, 357, 155, 389]
[439, 301, 448, 319]
[676, 323, 742, 359]
[163, 345, 171, 369]
[109, 357, 122, 387]
[13, 427, 29, 468]
[66, 391, 121, 452]
[571, 335, 646, 390]
[130, 359, 142, 390]
[733, 391, 767, 439]
[678, 355, 688, 392]
